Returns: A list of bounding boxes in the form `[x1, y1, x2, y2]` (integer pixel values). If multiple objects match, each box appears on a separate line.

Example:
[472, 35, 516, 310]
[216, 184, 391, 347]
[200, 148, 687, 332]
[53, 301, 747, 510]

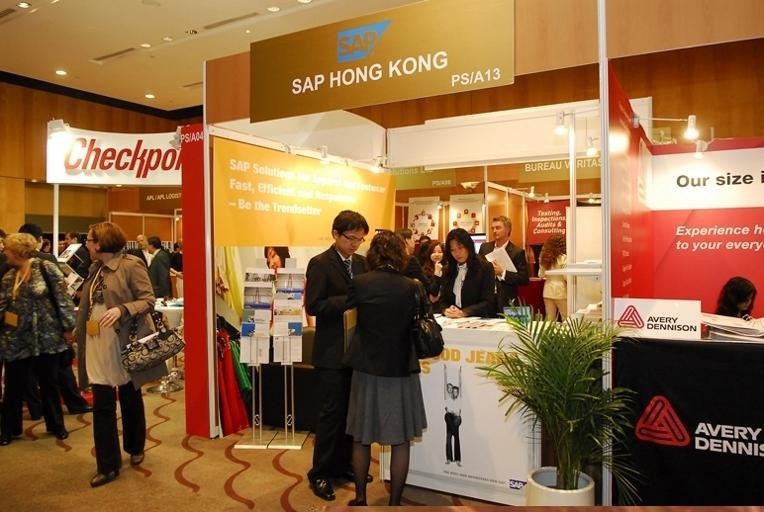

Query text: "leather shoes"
[131, 452, 144, 464]
[312, 477, 335, 501]
[344, 468, 372, 482]
[91, 470, 119, 487]
[2, 404, 94, 446]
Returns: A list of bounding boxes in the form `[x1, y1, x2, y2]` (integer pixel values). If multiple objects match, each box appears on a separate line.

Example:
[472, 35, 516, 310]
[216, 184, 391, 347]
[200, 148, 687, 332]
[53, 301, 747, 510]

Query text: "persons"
[75, 220, 156, 487]
[304, 209, 373, 500]
[0, 230, 184, 308]
[0, 233, 69, 446]
[18, 224, 92, 421]
[438, 228, 500, 318]
[396, 228, 448, 314]
[713, 276, 756, 322]
[445, 365, 462, 467]
[538, 236, 567, 322]
[342, 230, 434, 506]
[264, 246, 290, 269]
[479, 215, 529, 318]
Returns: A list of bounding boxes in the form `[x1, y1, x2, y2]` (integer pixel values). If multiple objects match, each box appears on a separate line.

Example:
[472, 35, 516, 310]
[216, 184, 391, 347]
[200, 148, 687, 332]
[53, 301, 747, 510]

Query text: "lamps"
[633, 114, 698, 140]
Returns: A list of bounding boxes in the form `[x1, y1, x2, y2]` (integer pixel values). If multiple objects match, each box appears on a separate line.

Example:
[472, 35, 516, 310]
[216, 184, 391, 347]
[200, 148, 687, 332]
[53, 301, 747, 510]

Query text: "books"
[700, 311, 764, 342]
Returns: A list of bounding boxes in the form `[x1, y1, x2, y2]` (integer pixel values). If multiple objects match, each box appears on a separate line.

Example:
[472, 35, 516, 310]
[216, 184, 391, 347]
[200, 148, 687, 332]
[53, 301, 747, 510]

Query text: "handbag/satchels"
[120, 326, 186, 373]
[413, 317, 444, 358]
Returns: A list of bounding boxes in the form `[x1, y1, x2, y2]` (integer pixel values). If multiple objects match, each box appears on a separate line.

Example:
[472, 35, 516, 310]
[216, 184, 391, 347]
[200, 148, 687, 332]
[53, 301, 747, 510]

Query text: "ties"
[344, 260, 351, 279]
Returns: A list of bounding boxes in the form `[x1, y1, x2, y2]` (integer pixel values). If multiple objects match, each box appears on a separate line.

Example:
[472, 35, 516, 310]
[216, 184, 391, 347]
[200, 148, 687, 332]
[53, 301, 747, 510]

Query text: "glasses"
[83, 238, 96, 244]
[340, 232, 365, 244]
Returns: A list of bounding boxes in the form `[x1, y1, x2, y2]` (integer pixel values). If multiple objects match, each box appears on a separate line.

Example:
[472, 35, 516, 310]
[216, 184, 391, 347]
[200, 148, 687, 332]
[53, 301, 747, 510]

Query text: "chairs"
[251, 327, 318, 432]
